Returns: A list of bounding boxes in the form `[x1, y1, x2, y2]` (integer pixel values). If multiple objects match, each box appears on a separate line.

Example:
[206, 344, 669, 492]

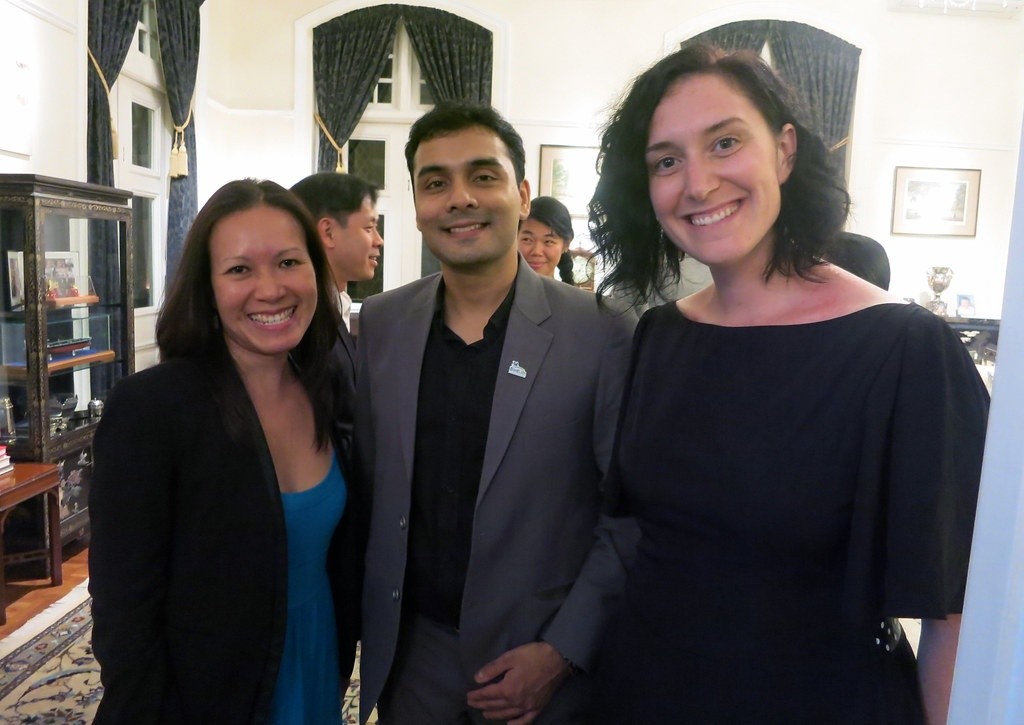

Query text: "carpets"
[0, 576, 379, 725]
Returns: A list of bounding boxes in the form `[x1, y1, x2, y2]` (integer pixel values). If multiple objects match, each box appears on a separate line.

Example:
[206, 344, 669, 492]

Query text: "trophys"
[923, 266, 954, 317]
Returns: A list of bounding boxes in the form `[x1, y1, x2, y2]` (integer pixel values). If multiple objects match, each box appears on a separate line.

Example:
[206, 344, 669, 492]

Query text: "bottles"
[0, 397, 15, 437]
[88, 399, 104, 421]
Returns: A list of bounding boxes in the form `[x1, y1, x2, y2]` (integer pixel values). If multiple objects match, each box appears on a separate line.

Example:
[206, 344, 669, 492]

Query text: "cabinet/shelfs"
[0, 173, 134, 576]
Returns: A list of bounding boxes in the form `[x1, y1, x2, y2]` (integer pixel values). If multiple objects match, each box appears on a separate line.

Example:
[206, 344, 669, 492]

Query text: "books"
[0, 445, 15, 475]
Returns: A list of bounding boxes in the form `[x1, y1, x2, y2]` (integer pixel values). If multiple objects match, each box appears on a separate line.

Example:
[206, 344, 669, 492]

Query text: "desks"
[0, 463, 62, 627]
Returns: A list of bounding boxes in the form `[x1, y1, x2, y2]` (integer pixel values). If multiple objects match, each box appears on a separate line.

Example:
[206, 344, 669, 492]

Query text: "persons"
[349, 102, 640, 725]
[956, 296, 975, 317]
[87, 180, 375, 725]
[514, 196, 576, 286]
[823, 231, 890, 291]
[595, 41, 992, 725]
[289, 172, 385, 338]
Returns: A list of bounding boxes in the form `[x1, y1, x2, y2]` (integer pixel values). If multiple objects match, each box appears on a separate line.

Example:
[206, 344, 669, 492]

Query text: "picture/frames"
[44, 251, 83, 298]
[891, 166, 982, 236]
[539, 144, 603, 221]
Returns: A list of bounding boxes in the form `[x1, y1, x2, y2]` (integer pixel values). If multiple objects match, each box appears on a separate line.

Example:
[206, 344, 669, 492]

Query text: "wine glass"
[925, 266, 954, 316]
